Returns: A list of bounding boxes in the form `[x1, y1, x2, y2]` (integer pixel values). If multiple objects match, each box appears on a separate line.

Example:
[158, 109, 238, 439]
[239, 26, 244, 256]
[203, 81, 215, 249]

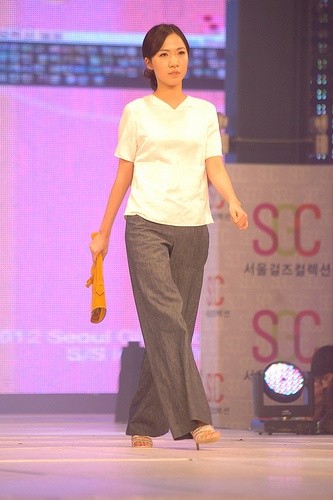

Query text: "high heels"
[131, 432, 153, 448]
[190, 422, 222, 450]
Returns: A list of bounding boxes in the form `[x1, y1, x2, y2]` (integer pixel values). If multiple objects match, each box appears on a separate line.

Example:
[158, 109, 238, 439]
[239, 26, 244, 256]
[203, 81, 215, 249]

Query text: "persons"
[91, 24, 248, 450]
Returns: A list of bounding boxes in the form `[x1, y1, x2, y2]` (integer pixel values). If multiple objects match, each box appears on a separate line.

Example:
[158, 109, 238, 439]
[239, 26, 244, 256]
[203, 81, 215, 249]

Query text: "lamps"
[253, 361, 315, 435]
